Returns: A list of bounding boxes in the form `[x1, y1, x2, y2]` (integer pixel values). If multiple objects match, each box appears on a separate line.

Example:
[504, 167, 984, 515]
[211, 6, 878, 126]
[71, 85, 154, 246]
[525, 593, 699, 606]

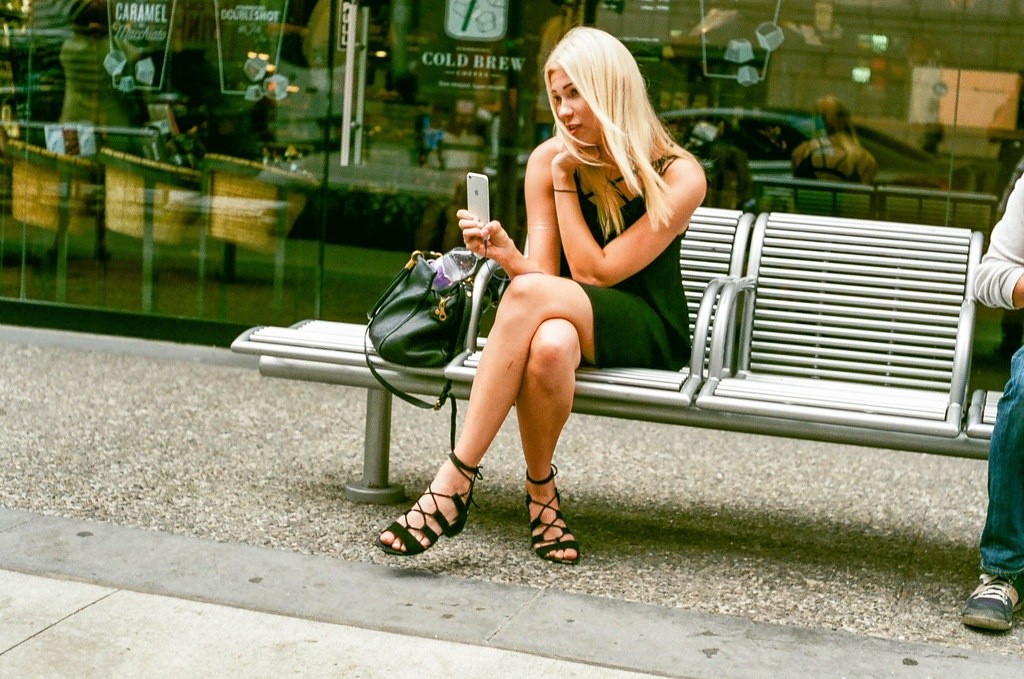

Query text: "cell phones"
[467, 172, 490, 240]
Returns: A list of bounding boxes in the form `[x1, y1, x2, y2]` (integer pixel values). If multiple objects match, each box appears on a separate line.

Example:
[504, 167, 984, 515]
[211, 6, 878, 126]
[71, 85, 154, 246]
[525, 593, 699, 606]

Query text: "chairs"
[199, 143, 333, 321]
[97, 143, 236, 315]
[4, 136, 106, 301]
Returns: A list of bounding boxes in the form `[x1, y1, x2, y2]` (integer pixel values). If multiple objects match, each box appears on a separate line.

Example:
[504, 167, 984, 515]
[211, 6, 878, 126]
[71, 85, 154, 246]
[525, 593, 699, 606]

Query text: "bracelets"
[553, 188, 577, 195]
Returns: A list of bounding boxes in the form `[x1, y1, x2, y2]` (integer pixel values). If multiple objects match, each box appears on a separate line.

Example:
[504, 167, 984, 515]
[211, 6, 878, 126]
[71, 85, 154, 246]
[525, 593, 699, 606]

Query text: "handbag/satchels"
[368, 249, 491, 368]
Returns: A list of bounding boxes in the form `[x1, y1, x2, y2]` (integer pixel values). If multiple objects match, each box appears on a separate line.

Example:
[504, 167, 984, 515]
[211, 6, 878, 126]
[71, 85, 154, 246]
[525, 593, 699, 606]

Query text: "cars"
[482, 106, 961, 258]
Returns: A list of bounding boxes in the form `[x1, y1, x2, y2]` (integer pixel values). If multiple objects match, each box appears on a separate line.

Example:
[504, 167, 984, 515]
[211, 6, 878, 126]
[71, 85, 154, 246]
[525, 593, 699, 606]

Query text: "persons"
[412, 100, 451, 172]
[964, 170, 1024, 635]
[51, 7, 131, 261]
[376, 25, 707, 564]
[682, 93, 875, 217]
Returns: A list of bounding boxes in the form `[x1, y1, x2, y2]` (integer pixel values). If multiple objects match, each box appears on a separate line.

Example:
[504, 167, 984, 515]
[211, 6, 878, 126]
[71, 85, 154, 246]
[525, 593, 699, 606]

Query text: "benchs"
[741, 166, 1000, 235]
[230, 201, 1008, 508]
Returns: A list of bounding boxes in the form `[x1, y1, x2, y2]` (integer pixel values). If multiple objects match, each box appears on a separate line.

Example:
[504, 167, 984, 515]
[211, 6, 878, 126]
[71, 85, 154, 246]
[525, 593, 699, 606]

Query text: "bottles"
[429, 246, 484, 293]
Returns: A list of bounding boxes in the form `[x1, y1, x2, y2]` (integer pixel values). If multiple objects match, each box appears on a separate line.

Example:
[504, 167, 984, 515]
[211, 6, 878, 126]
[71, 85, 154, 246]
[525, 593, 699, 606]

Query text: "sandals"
[374, 452, 484, 555]
[525, 463, 582, 565]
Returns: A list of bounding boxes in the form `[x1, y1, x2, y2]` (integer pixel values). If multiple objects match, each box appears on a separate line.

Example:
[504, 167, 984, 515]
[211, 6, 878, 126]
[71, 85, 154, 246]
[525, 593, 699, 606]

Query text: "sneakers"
[960, 573, 1024, 629]
